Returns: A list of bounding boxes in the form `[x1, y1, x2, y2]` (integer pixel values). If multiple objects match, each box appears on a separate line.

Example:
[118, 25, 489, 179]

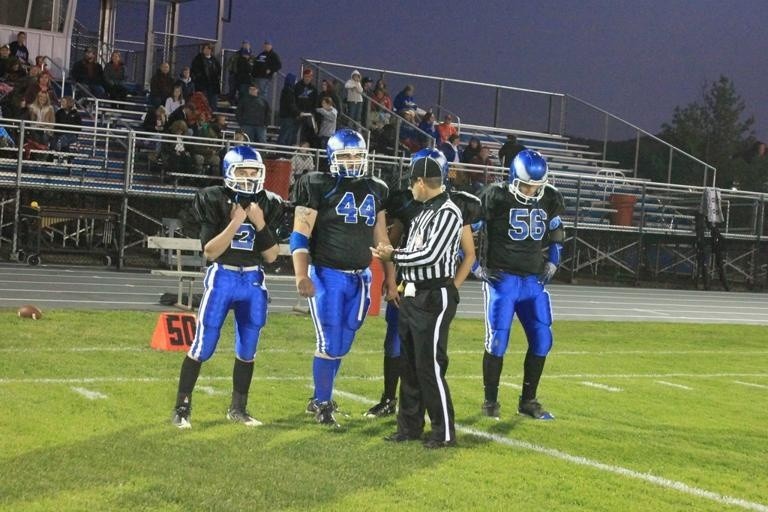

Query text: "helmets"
[508, 149, 549, 205]
[223, 145, 265, 195]
[410, 148, 448, 179]
[327, 128, 366, 179]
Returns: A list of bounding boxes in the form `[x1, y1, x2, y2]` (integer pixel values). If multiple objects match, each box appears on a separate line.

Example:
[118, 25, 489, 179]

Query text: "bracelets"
[255, 224, 275, 252]
[289, 231, 310, 256]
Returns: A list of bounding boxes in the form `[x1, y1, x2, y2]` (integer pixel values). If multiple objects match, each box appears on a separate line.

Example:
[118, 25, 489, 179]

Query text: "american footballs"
[19, 304, 41, 320]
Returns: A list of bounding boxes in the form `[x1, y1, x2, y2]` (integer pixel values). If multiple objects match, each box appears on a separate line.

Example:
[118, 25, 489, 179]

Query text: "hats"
[412, 157, 442, 177]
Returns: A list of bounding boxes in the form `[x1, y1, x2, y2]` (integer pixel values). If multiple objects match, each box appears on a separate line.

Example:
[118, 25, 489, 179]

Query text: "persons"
[368, 158, 461, 449]
[474, 149, 566, 419]
[365, 149, 484, 417]
[170, 145, 285, 429]
[290, 127, 400, 426]
[1, 28, 528, 190]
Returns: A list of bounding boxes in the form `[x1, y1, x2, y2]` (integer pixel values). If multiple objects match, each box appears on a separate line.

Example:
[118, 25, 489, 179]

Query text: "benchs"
[0, 96, 285, 186]
[435, 121, 696, 233]
[146, 237, 317, 314]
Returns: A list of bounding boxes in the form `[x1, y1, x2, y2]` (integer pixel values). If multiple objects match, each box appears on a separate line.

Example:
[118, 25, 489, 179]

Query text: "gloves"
[537, 262, 558, 284]
[476, 267, 503, 287]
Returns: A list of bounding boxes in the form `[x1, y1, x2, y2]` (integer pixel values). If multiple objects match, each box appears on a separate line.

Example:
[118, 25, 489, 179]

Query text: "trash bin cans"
[609, 194, 637, 226]
[263, 159, 292, 201]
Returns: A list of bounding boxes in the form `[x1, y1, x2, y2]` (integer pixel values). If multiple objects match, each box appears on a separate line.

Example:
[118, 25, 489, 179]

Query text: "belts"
[338, 267, 366, 273]
[222, 264, 259, 272]
